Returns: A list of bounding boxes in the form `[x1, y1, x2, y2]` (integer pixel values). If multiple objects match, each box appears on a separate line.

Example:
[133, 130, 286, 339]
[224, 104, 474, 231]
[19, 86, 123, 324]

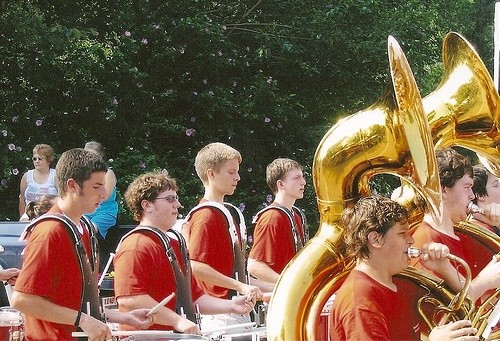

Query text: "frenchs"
[266, 35, 500, 341]
[391, 32, 500, 341]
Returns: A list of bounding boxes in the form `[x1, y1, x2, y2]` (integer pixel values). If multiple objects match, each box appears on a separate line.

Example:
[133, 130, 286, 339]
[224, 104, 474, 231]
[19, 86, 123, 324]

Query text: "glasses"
[152, 195, 179, 203]
[31, 157, 47, 161]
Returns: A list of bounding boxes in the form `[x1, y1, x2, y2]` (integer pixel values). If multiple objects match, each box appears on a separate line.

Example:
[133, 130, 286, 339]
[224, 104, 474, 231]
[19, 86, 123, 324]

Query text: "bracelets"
[74, 310, 81, 327]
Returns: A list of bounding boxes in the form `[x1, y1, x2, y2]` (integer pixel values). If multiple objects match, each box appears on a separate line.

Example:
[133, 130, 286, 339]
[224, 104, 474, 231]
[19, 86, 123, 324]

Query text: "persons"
[83, 141, 118, 240]
[112, 169, 203, 341]
[326, 194, 479, 341]
[409, 147, 500, 309]
[10, 148, 116, 341]
[18, 144, 59, 223]
[182, 142, 275, 341]
[469, 164, 500, 235]
[247, 158, 310, 285]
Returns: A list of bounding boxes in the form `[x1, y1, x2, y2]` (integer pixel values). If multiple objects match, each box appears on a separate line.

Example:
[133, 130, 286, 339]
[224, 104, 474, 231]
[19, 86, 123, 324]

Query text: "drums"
[0, 307, 23, 341]
[218, 327, 267, 341]
[121, 334, 210, 341]
